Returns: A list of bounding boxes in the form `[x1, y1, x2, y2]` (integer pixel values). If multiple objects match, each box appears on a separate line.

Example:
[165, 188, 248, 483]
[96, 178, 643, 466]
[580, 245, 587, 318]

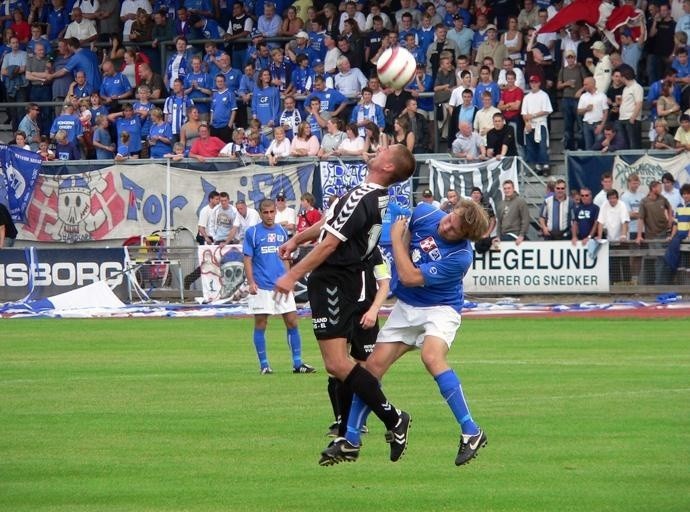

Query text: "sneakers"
[292, 365, 317, 374]
[456, 430, 488, 466]
[385, 411, 412, 462]
[320, 425, 369, 467]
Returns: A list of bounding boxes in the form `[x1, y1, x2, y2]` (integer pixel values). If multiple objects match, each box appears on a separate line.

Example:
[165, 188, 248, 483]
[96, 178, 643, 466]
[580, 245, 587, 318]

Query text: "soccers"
[376, 48, 416, 89]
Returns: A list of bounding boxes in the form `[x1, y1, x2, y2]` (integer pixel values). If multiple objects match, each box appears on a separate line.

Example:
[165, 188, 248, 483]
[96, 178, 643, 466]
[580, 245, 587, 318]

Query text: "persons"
[327, 245, 393, 436]
[1, 2, 690, 176]
[0, 203, 18, 248]
[182, 191, 321, 290]
[272, 144, 418, 462]
[321, 196, 489, 466]
[241, 200, 316, 374]
[422, 172, 690, 286]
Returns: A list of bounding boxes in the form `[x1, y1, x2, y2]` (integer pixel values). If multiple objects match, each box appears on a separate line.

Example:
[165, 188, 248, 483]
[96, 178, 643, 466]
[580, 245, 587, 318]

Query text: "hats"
[530, 76, 539, 82]
[454, 14, 464, 20]
[423, 189, 432, 195]
[276, 193, 285, 198]
[251, 29, 261, 38]
[591, 41, 604, 50]
[294, 33, 309, 39]
[566, 50, 575, 57]
